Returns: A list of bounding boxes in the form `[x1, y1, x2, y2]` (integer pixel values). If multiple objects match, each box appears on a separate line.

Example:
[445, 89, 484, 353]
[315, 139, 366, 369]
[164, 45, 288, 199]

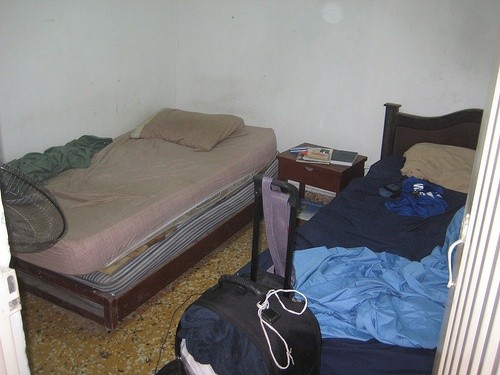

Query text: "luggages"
[174, 173, 323, 375]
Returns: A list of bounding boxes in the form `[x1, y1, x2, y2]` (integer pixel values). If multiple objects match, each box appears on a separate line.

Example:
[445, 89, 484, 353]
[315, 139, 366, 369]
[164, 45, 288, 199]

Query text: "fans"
[1, 163, 68, 254]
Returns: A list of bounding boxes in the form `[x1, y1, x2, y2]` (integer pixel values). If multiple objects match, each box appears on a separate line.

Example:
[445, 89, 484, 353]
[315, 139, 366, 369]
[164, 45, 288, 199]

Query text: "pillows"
[128, 108, 248, 152]
[400, 142, 476, 193]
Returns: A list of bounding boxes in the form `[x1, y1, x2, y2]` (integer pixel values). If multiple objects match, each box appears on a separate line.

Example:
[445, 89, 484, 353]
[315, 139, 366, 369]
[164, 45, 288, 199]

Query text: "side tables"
[276, 143, 368, 200]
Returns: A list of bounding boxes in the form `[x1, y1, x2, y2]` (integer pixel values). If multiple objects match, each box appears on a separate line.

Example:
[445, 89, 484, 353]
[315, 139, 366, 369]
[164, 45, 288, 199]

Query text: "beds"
[0, 125, 280, 330]
[175, 103, 485, 375]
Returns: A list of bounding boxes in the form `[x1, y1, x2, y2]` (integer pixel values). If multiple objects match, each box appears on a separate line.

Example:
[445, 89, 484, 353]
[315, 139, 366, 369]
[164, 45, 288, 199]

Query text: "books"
[330, 150, 358, 167]
[289, 146, 333, 165]
